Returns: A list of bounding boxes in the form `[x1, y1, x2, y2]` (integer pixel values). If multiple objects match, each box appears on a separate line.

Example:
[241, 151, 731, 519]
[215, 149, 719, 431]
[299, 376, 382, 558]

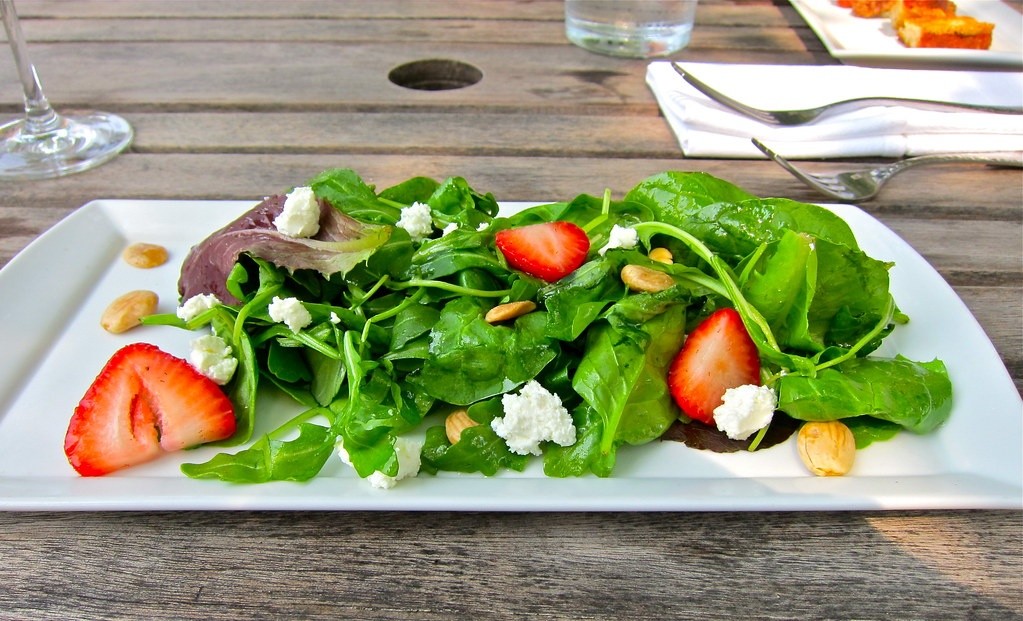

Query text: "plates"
[790, 0, 1022, 66]
[2, 199, 1023, 513]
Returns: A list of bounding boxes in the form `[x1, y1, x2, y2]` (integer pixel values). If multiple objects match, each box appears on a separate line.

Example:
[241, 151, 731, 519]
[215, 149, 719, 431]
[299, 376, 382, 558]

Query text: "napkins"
[644, 59, 1023, 168]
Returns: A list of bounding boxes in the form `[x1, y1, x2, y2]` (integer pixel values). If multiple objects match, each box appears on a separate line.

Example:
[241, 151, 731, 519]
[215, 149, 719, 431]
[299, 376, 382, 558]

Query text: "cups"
[561, 1, 698, 59]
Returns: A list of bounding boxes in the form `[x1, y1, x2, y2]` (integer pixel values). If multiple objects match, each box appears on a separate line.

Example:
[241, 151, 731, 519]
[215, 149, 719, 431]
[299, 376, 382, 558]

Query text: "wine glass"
[0, 0, 135, 181]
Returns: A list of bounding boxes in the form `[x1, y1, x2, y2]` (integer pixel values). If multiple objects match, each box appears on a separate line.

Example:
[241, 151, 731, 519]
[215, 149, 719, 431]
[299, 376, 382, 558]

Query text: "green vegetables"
[142, 163, 954, 487]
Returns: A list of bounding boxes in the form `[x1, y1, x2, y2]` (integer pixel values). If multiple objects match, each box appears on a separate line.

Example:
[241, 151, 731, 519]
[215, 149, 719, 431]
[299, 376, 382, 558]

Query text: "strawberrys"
[63, 343, 235, 478]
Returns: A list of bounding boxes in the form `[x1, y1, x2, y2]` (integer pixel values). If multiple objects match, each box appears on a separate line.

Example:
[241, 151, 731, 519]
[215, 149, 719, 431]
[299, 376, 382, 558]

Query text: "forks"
[669, 60, 1023, 130]
[751, 136, 1023, 203]
